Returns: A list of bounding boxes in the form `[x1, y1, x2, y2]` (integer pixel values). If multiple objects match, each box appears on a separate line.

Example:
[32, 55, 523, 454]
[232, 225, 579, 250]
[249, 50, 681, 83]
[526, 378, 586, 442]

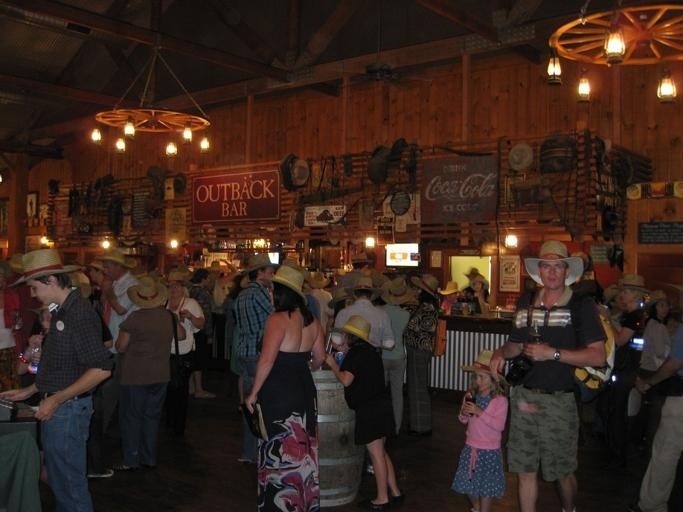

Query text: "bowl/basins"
[329, 331, 344, 345]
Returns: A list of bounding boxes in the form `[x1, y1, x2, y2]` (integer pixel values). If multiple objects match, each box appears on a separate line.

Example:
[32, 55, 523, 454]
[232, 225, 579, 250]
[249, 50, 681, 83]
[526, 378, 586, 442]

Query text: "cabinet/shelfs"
[59, 247, 156, 272]
[625, 195, 683, 283]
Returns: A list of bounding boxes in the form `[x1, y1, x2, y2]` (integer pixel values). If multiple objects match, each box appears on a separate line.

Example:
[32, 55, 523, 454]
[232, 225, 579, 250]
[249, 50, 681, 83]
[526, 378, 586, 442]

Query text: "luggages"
[157, 376, 189, 459]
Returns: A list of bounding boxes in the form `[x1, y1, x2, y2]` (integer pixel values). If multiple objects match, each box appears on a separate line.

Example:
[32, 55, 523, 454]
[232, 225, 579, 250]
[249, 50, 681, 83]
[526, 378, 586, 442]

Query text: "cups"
[178, 309, 186, 323]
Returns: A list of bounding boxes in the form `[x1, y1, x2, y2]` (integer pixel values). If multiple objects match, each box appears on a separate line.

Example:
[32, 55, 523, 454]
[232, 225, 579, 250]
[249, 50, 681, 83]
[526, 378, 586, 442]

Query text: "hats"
[240, 254, 280, 275]
[410, 274, 440, 299]
[127, 277, 167, 309]
[70, 272, 91, 298]
[603, 274, 683, 310]
[332, 315, 375, 347]
[205, 261, 229, 272]
[160, 271, 192, 288]
[461, 349, 510, 385]
[309, 272, 330, 289]
[76, 251, 137, 271]
[465, 268, 489, 291]
[269, 265, 305, 299]
[380, 278, 416, 305]
[7, 248, 84, 287]
[523, 240, 584, 288]
[438, 281, 461, 296]
[327, 278, 384, 309]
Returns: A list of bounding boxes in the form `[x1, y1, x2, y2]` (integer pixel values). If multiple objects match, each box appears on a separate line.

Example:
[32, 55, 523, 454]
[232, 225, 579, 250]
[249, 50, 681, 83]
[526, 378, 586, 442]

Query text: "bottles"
[218, 240, 272, 252]
[16, 344, 41, 375]
[464, 389, 474, 417]
[529, 320, 546, 367]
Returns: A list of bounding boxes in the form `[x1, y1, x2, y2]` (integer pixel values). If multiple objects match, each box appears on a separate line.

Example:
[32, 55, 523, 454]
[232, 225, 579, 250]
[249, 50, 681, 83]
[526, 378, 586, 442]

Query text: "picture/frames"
[25, 191, 38, 230]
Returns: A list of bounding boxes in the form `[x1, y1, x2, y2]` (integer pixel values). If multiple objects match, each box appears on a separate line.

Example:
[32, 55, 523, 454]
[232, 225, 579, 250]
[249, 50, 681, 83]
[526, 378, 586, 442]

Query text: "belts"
[38, 390, 90, 400]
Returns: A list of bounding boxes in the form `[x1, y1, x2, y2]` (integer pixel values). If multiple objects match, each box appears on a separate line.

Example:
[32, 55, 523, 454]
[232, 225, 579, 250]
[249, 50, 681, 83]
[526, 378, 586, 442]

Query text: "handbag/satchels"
[86, 436, 113, 470]
[173, 346, 202, 375]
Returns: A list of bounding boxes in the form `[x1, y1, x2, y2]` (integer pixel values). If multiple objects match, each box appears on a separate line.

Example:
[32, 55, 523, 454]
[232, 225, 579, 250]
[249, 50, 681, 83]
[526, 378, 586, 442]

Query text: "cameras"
[505, 356, 532, 386]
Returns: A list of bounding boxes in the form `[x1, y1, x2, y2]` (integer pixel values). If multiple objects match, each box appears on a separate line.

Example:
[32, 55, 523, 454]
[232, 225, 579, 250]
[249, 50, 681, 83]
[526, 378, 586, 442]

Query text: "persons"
[1, 242, 683, 512]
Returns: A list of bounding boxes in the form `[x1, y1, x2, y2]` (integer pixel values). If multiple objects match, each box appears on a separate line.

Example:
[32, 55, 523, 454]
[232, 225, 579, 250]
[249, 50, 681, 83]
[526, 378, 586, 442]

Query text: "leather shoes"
[194, 389, 216, 399]
[360, 489, 404, 512]
[405, 429, 432, 441]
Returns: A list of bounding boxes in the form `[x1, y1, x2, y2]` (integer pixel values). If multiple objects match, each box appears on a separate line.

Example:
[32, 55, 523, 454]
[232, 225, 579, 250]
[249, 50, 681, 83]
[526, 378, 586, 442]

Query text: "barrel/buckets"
[310, 369, 365, 508]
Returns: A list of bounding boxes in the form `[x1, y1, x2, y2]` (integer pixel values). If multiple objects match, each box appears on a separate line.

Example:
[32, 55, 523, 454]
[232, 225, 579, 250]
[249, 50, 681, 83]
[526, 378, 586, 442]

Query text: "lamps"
[253, 238, 267, 249]
[90, 48, 212, 157]
[505, 233, 518, 249]
[365, 235, 376, 249]
[545, 1, 683, 105]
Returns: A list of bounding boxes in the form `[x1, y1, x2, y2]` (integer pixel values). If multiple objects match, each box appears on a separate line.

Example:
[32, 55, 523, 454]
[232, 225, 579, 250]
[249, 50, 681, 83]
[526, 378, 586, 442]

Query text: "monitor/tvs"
[254, 252, 279, 264]
[385, 242, 421, 268]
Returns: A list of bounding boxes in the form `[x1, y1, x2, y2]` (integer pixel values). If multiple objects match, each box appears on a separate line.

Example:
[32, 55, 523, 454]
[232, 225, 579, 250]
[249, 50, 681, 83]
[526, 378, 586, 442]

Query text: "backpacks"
[576, 302, 616, 402]
